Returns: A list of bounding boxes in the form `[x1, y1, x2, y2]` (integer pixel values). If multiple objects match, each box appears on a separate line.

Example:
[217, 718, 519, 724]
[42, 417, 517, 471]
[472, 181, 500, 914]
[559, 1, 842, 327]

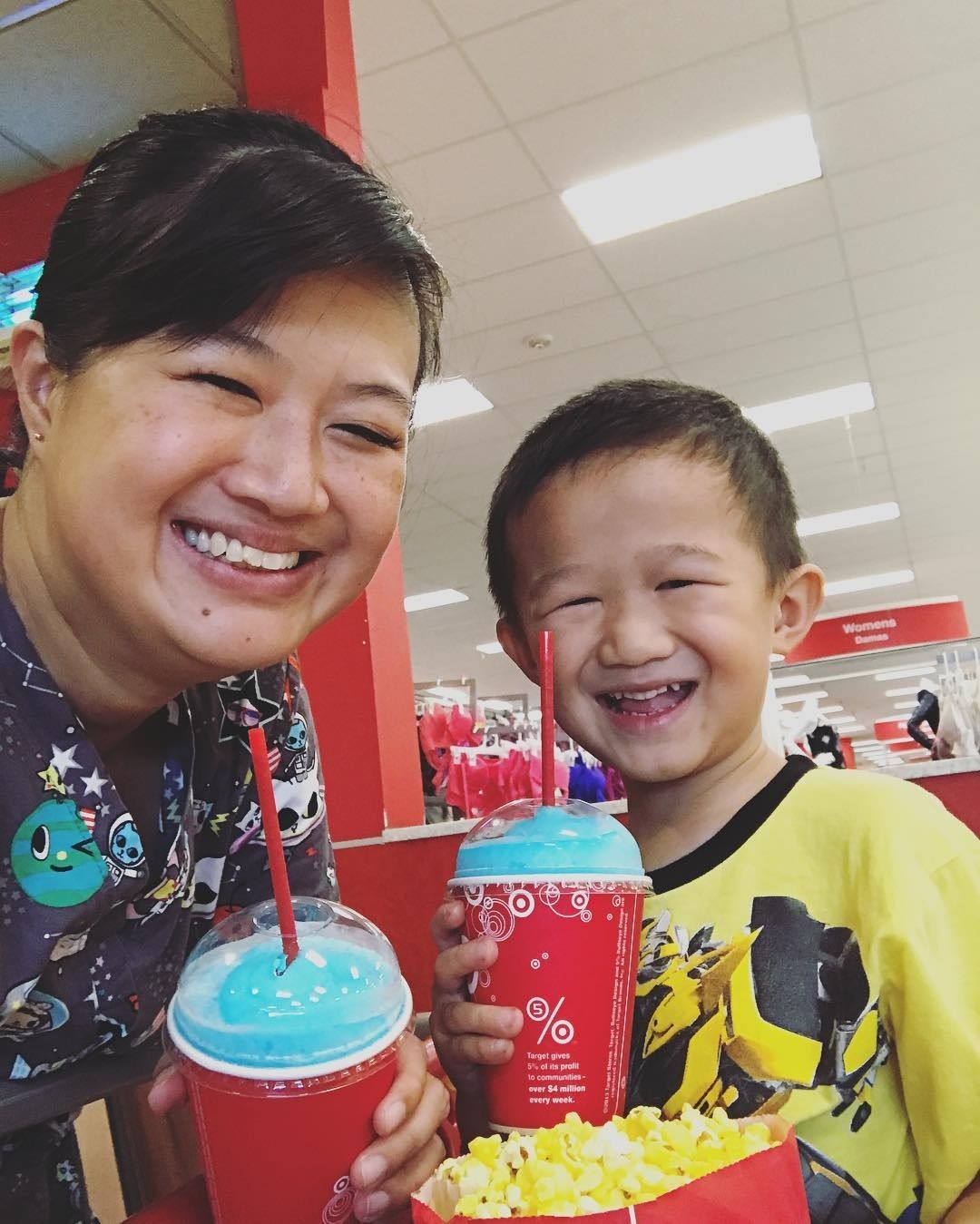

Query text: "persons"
[787, 698, 846, 771]
[430, 381, 978, 1224]
[0, 106, 456, 1224]
[907, 677, 952, 762]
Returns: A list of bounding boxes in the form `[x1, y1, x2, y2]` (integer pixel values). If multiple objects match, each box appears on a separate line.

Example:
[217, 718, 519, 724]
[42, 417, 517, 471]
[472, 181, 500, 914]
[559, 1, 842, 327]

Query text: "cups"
[157, 893, 417, 1224]
[444, 794, 656, 1137]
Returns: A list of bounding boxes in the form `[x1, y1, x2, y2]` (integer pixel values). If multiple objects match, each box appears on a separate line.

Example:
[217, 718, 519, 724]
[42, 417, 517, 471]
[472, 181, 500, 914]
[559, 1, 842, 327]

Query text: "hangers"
[448, 712, 607, 772]
[940, 647, 979, 696]
[421, 676, 478, 719]
